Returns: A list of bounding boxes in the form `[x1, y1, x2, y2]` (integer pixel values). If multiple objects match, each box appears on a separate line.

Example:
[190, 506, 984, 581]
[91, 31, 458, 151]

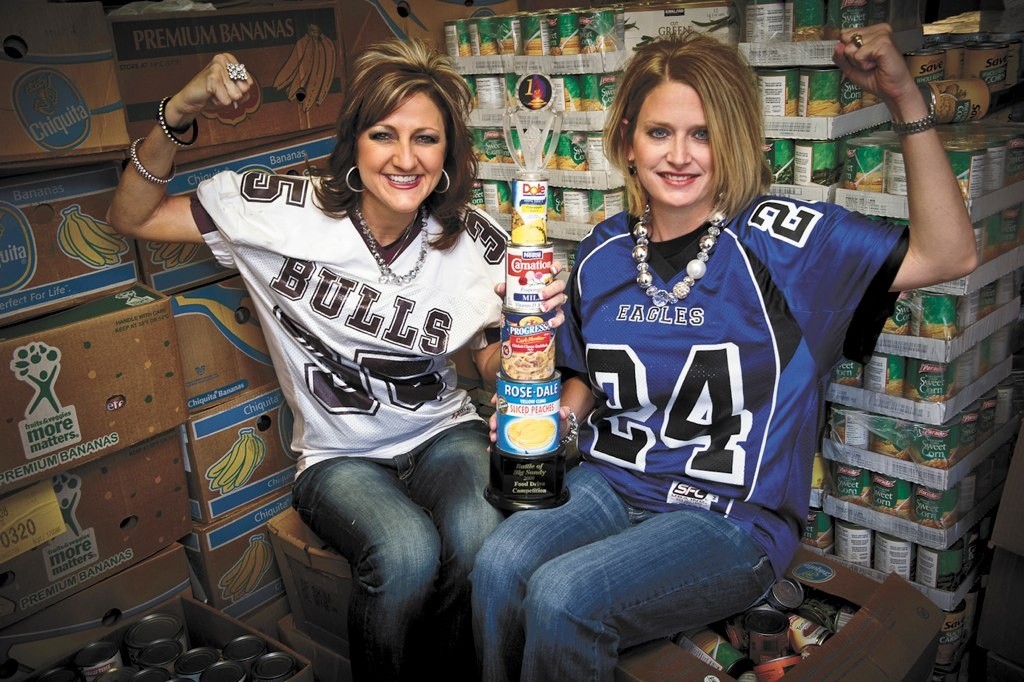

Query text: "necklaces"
[388, 210, 418, 266]
[632, 193, 729, 308]
[352, 202, 429, 287]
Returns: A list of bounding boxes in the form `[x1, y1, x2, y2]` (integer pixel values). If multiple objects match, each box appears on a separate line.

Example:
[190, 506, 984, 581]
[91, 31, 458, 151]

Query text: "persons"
[106, 38, 567, 682]
[468, 24, 979, 682]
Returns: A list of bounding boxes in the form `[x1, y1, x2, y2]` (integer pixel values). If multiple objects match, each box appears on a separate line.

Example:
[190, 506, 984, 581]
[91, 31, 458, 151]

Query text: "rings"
[561, 292, 568, 305]
[851, 33, 864, 50]
[226, 62, 248, 82]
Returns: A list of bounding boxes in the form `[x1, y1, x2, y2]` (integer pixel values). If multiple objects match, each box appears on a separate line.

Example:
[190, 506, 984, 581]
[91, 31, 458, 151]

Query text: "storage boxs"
[0, 0, 1024, 682]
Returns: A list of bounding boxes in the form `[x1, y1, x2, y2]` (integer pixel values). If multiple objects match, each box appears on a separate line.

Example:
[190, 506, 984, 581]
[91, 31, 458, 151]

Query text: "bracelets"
[159, 96, 201, 149]
[561, 411, 578, 445]
[130, 136, 177, 185]
[155, 111, 192, 134]
[893, 103, 939, 135]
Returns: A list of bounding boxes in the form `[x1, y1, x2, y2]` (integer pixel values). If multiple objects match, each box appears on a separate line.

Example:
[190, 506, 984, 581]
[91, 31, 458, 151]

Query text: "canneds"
[438, 0, 1024, 682]
[34, 614, 299, 682]
